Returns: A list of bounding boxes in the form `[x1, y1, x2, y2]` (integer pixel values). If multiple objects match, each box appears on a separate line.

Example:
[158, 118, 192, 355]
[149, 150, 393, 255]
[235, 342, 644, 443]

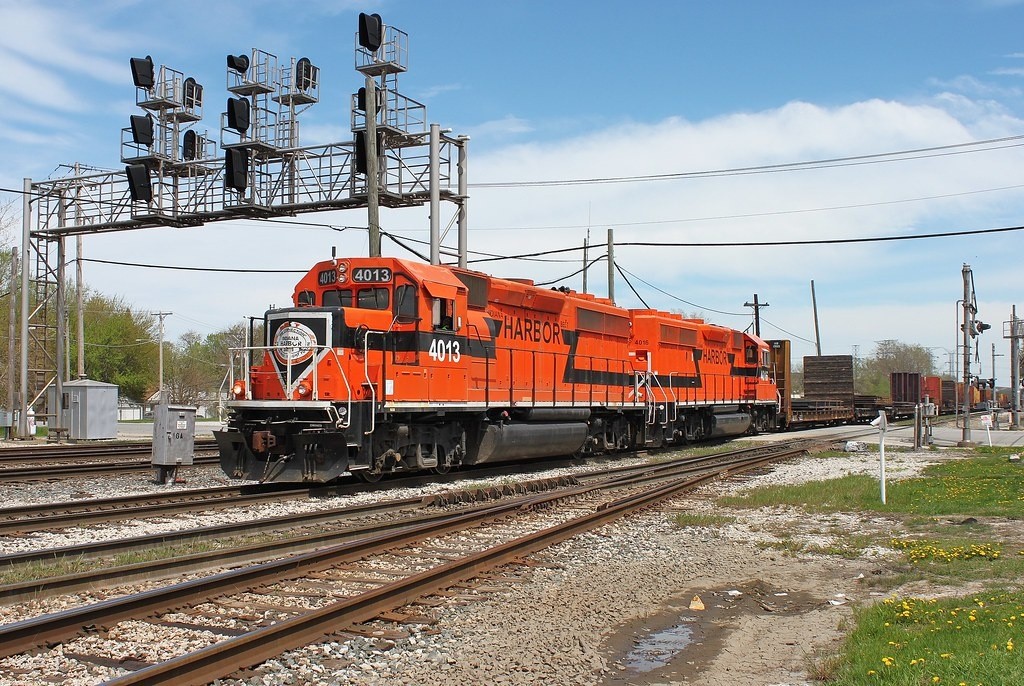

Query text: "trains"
[213, 244, 1010, 494]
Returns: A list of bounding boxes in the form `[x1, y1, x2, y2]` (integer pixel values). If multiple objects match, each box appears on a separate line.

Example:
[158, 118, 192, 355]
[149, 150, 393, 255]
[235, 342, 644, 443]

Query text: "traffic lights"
[358, 12, 382, 52]
[227, 97, 249, 131]
[130, 114, 153, 147]
[129, 55, 154, 88]
[126, 162, 152, 203]
[227, 53, 251, 73]
[225, 146, 249, 192]
